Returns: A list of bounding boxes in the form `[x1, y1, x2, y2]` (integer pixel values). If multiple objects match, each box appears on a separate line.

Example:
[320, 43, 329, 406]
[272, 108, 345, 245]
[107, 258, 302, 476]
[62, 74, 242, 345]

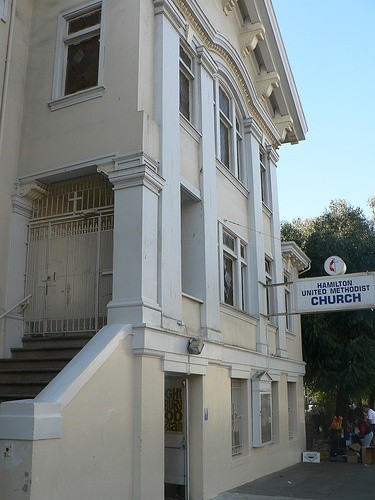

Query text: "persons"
[326, 404, 375, 460]
